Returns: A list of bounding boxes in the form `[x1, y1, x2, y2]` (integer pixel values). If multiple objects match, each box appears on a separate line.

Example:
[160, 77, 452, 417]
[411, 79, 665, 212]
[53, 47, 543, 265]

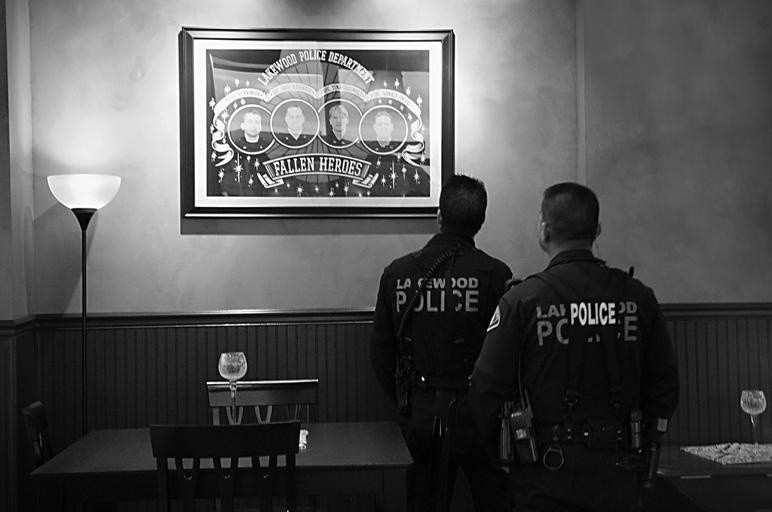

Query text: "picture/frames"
[180, 26, 455, 220]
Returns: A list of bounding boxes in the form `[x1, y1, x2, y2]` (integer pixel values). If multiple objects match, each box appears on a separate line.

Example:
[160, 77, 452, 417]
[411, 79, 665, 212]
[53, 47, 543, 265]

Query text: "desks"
[651, 443, 772, 512]
[29, 421, 414, 512]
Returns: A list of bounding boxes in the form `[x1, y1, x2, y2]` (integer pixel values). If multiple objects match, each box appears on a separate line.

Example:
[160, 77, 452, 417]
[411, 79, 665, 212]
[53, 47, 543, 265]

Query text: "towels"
[680, 442, 772, 465]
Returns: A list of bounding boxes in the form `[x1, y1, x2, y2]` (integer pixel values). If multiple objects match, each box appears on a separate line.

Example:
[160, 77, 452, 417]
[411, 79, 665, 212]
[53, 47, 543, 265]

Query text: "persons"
[470, 181, 678, 511]
[367, 112, 398, 152]
[278, 106, 313, 146]
[324, 105, 353, 146]
[370, 174, 513, 512]
[233, 111, 269, 151]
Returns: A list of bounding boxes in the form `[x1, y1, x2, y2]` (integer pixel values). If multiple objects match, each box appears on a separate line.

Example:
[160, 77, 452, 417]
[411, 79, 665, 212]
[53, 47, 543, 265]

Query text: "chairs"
[206, 379, 319, 424]
[23, 401, 54, 470]
[149, 420, 301, 512]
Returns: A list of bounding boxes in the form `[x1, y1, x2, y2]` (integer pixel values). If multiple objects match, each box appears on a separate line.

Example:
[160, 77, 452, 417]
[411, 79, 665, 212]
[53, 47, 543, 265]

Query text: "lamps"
[47, 174, 122, 436]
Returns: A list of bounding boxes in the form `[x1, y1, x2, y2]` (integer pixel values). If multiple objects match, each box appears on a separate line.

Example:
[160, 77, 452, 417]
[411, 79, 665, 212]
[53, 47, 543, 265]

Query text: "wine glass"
[217, 351, 249, 424]
[740, 390, 768, 456]
